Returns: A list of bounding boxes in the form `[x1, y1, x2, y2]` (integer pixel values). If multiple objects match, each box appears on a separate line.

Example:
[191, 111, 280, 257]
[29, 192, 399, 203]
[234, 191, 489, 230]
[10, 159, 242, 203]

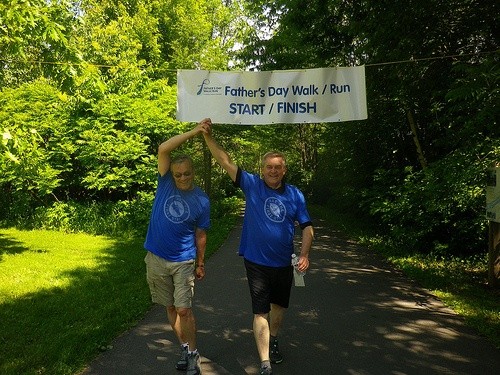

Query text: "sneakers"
[174, 343, 201, 375]
[270, 336, 283, 364]
[258, 364, 273, 375]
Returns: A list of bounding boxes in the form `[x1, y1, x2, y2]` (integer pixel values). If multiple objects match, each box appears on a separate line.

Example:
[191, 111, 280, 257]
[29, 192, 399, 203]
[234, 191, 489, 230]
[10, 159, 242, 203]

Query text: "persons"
[143, 118, 213, 375]
[202, 118, 315, 375]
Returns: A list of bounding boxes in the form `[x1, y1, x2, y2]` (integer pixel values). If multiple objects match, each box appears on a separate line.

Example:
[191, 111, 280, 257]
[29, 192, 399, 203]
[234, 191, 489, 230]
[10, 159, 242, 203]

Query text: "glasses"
[174, 172, 192, 178]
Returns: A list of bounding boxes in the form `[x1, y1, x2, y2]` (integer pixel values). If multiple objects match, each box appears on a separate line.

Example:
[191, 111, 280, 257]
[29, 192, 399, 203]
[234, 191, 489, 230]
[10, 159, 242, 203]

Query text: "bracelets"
[197, 264, 204, 267]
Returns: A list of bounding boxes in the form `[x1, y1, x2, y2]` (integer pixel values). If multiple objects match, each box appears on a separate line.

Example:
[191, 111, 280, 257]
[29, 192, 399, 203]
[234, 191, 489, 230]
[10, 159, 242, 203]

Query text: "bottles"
[291, 253, 306, 277]
